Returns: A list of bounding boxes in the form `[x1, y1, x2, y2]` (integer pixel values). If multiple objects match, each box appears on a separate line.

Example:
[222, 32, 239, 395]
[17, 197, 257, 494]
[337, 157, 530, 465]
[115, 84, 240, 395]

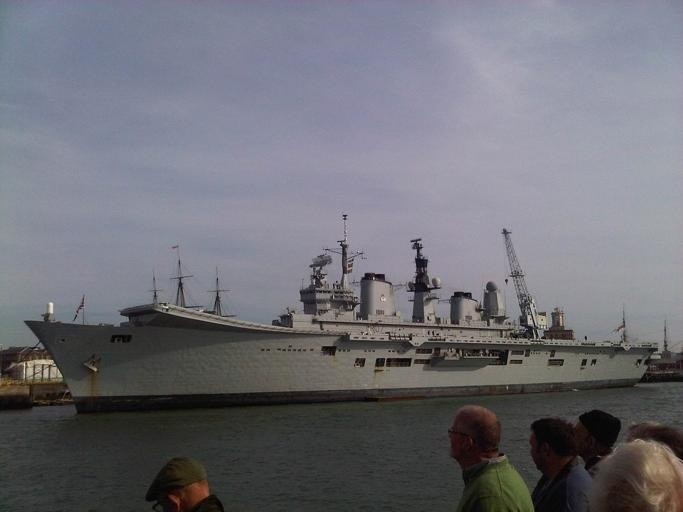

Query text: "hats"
[578, 409, 622, 447]
[145, 455, 208, 502]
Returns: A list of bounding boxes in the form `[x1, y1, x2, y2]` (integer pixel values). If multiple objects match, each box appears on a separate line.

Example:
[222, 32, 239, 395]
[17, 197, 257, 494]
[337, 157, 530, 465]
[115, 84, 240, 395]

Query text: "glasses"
[152, 502, 164, 512]
[448, 427, 469, 436]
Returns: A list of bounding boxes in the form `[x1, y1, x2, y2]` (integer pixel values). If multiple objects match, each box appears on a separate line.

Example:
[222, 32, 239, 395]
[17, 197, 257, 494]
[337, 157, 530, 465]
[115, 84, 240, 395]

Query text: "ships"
[23, 214, 659, 414]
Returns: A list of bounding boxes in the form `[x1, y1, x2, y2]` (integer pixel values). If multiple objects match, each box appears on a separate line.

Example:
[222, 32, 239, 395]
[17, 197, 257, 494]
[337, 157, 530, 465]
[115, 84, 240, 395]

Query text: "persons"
[447, 404, 536, 512]
[580, 435, 683, 512]
[529, 416, 594, 512]
[145, 456, 225, 512]
[626, 417, 683, 463]
[571, 408, 622, 477]
